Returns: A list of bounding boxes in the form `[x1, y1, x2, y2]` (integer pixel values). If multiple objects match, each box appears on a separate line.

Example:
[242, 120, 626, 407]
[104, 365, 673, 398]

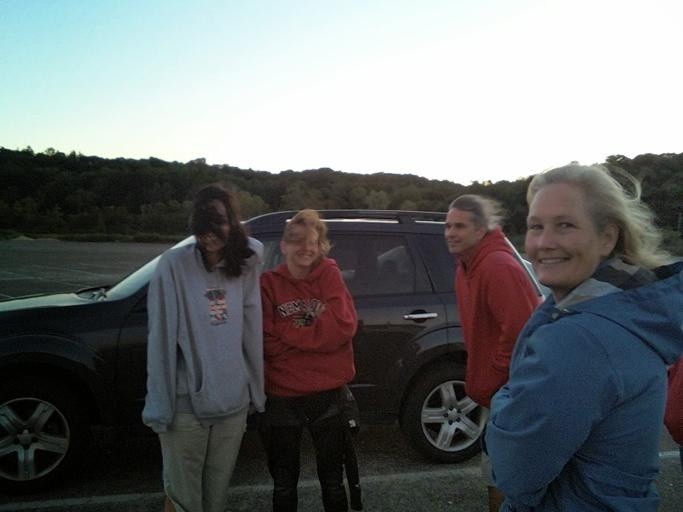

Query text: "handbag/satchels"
[343, 385, 360, 436]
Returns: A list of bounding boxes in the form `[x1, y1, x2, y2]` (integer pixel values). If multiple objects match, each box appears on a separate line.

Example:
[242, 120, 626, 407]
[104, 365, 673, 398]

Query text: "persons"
[482, 164, 683, 511]
[142, 184, 268, 512]
[444, 193, 541, 512]
[259, 209, 358, 512]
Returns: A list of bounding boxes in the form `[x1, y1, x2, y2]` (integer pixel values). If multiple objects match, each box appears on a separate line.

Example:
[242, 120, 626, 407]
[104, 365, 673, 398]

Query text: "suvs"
[0, 207, 546, 496]
